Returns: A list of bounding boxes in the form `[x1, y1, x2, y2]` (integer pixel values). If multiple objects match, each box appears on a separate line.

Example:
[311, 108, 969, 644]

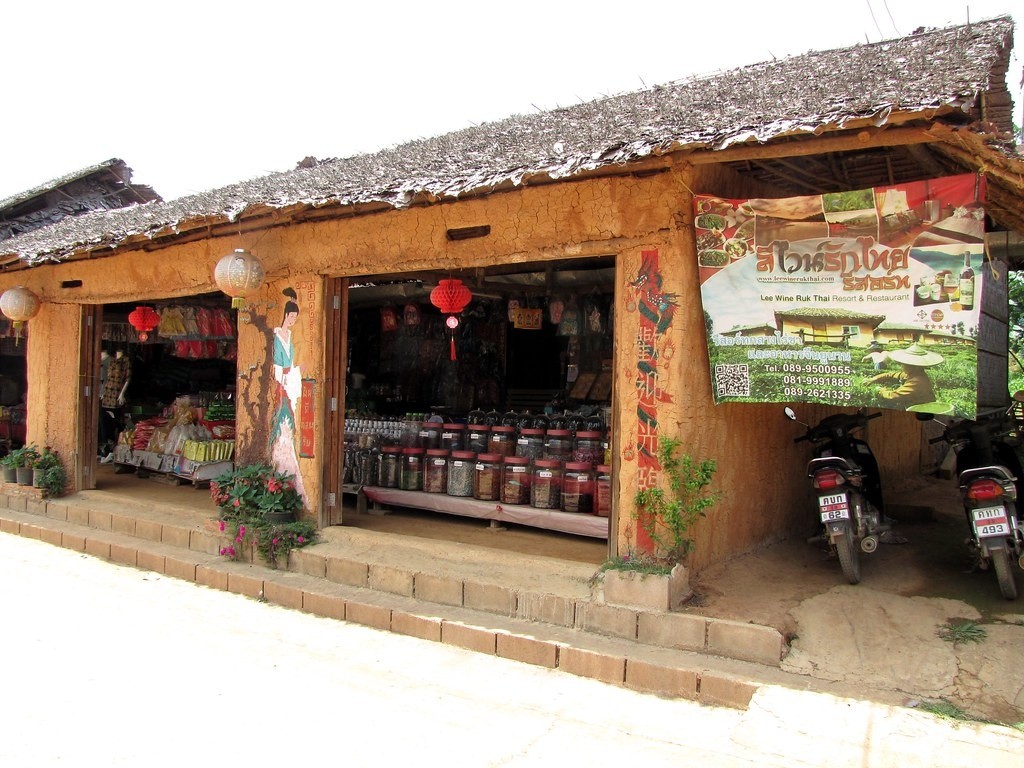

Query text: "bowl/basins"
[916, 286, 930, 299]
[701, 202, 711, 211]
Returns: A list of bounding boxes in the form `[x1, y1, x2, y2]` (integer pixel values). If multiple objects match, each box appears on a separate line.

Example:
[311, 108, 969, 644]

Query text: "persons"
[97, 350, 132, 463]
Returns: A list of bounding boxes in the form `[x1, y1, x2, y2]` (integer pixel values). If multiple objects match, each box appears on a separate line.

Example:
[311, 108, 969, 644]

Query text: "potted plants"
[0, 450, 19, 483]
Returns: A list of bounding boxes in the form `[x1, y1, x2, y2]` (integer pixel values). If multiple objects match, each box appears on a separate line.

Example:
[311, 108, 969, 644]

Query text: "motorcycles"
[913, 388, 1024, 601]
[784, 404, 893, 586]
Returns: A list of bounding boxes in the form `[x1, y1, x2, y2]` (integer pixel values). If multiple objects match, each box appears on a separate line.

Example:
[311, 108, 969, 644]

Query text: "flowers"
[15, 443, 42, 466]
[585, 548, 669, 585]
[34, 445, 69, 495]
[209, 462, 316, 563]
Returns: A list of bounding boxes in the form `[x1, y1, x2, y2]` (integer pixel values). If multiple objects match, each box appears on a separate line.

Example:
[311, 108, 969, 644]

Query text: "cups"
[931, 284, 941, 300]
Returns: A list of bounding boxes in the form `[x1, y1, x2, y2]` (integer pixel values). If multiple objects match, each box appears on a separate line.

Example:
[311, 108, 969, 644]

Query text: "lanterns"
[429, 278, 472, 361]
[0, 284, 41, 347]
[127, 306, 161, 343]
[214, 248, 266, 309]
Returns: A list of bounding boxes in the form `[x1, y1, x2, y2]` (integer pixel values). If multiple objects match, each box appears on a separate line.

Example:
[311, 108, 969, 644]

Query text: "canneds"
[343, 418, 404, 438]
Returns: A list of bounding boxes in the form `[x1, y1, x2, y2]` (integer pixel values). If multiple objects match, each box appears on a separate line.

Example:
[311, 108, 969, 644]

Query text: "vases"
[16, 467, 33, 486]
[603, 564, 693, 611]
[33, 468, 45, 487]
[258, 510, 293, 524]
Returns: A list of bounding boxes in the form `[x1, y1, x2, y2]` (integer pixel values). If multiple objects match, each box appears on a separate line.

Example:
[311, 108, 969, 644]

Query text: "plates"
[694, 214, 727, 231]
[697, 249, 731, 268]
[723, 238, 748, 259]
[696, 230, 726, 251]
[738, 202, 755, 217]
[732, 217, 755, 242]
[710, 199, 733, 216]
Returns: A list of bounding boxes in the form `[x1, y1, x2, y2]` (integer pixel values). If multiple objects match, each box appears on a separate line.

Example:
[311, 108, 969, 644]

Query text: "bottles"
[959, 251, 975, 310]
[401, 413, 425, 447]
[418, 422, 611, 465]
[344, 446, 611, 516]
[344, 416, 406, 438]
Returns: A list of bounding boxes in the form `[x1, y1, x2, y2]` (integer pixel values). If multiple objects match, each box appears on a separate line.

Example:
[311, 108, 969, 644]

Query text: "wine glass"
[944, 274, 959, 305]
